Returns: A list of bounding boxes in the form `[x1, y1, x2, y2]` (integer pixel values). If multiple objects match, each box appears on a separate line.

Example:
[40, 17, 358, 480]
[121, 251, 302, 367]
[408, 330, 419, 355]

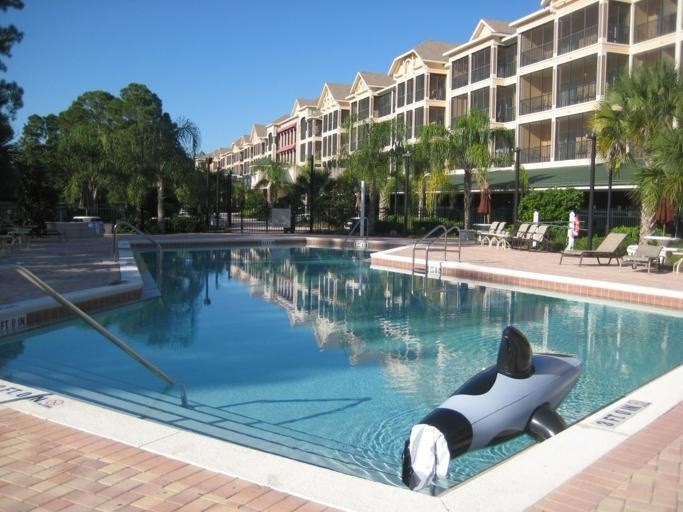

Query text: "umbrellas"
[478, 179, 491, 224]
[655, 191, 676, 238]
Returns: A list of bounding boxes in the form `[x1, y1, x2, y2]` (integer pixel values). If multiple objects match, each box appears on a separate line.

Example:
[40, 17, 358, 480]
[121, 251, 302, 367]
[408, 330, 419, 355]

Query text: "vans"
[74, 216, 105, 232]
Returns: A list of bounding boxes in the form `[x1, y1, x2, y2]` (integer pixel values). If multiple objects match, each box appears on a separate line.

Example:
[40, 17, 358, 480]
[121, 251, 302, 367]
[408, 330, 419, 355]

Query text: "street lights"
[510, 146, 520, 235]
[214, 164, 222, 227]
[606, 146, 619, 236]
[585, 131, 597, 251]
[204, 156, 211, 227]
[214, 252, 219, 290]
[308, 154, 314, 232]
[203, 265, 211, 306]
[225, 169, 235, 226]
[402, 151, 413, 236]
[227, 250, 232, 280]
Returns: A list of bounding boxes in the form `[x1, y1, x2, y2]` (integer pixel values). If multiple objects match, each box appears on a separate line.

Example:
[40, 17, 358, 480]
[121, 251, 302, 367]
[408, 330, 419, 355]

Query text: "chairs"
[0, 225, 67, 255]
[473, 218, 681, 273]
